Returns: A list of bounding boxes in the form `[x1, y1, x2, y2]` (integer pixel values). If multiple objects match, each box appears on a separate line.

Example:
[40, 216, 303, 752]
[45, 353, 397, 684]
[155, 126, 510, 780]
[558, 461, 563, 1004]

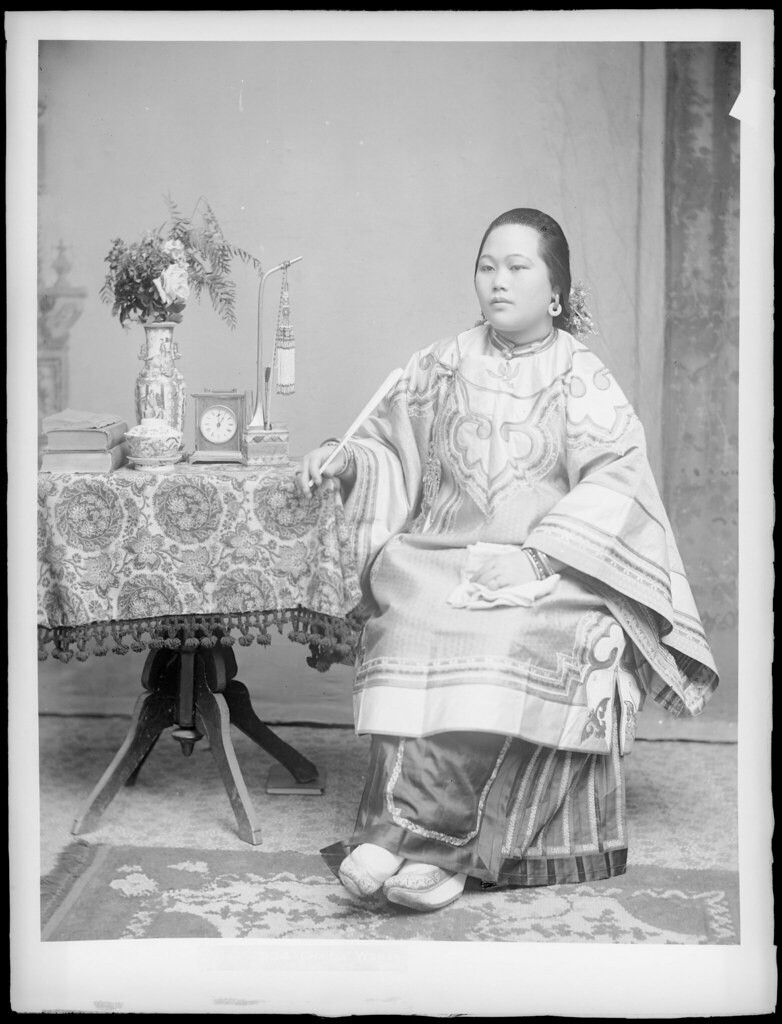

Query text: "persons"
[303, 208, 720, 910]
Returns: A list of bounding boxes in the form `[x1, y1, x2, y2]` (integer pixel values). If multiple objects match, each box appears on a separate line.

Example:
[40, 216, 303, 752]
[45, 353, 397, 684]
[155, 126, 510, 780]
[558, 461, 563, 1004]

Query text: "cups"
[123, 419, 184, 457]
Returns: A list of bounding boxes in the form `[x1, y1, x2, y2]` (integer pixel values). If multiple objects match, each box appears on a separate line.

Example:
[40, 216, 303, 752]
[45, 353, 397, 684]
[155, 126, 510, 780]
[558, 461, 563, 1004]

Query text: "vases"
[135, 323, 185, 435]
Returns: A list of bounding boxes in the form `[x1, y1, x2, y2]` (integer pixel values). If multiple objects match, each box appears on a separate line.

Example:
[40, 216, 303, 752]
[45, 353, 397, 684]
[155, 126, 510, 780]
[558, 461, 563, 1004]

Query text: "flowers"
[99, 188, 266, 329]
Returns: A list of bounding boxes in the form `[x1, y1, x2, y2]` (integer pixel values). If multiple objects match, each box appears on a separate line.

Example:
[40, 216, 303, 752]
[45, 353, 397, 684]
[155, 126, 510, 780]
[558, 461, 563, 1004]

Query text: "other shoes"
[381, 868, 467, 909]
[339, 843, 402, 896]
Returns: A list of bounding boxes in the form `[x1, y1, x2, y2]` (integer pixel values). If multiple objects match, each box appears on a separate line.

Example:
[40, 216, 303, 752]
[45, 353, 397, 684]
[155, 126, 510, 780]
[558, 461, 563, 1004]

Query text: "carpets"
[37, 837, 741, 944]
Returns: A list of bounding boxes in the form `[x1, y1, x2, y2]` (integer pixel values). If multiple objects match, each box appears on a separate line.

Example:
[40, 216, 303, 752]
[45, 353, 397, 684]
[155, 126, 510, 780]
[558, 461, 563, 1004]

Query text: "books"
[39, 407, 131, 474]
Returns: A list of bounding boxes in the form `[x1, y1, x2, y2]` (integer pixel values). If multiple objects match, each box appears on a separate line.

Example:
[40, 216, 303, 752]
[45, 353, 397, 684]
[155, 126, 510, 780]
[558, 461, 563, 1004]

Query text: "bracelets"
[318, 437, 354, 478]
[523, 548, 554, 582]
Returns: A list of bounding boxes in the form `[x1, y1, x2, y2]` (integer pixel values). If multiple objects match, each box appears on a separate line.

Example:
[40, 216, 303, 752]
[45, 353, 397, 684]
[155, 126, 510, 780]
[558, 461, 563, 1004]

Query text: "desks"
[37, 462, 362, 846]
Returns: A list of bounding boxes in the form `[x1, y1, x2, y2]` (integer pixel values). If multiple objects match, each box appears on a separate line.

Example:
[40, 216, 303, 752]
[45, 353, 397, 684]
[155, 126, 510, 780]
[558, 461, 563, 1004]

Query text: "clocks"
[191, 387, 247, 465]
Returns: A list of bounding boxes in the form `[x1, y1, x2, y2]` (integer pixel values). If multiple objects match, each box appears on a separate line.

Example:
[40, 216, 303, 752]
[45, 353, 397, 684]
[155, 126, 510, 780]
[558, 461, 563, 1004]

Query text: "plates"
[127, 454, 182, 472]
